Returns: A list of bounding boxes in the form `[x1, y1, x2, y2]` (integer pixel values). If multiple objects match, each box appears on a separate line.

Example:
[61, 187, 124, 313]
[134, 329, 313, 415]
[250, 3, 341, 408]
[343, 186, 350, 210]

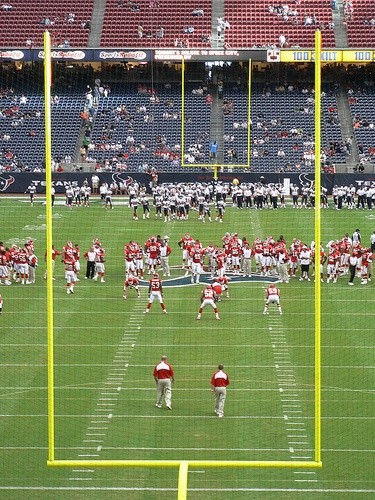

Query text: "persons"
[142, 273, 167, 314]
[0, 239, 106, 295]
[124, 229, 374, 287]
[196, 284, 220, 320]
[123, 275, 141, 299]
[210, 364, 230, 417]
[0, 59, 374, 223]
[152, 355, 174, 411]
[263, 284, 281, 316]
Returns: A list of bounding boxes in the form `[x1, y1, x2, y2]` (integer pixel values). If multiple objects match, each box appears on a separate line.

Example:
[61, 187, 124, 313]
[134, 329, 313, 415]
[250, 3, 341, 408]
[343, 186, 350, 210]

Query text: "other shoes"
[146, 310, 167, 314]
[196, 317, 221, 320]
[156, 403, 162, 408]
[215, 409, 224, 418]
[0, 266, 375, 302]
[263, 312, 282, 315]
[166, 403, 172, 410]
